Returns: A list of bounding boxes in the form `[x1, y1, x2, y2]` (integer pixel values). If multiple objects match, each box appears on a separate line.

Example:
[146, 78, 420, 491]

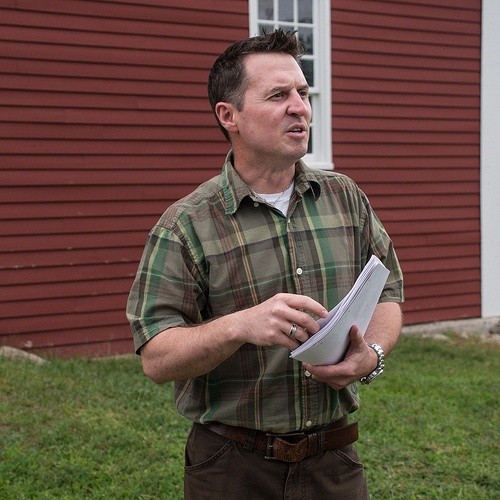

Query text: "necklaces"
[270, 180, 294, 206]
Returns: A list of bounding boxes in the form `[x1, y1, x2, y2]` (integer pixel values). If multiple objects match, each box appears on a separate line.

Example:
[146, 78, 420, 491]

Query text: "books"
[290, 255, 391, 367]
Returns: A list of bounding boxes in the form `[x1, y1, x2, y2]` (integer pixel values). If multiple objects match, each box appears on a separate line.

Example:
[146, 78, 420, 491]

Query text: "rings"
[289, 323, 296, 338]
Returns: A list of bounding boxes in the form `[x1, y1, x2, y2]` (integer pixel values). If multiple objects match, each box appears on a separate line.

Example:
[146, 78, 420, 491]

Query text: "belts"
[201, 414, 359, 462]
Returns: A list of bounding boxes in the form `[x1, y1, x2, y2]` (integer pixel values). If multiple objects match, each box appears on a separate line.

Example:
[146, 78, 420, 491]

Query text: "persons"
[124, 24, 405, 500]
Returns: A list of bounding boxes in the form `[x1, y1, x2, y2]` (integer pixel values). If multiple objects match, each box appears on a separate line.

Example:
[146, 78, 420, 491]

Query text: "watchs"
[359, 342, 385, 386]
